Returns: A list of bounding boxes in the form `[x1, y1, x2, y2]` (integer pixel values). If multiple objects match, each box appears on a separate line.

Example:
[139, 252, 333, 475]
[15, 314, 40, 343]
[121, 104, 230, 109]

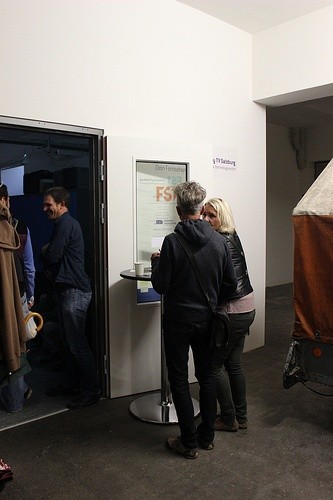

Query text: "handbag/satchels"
[209, 310, 233, 351]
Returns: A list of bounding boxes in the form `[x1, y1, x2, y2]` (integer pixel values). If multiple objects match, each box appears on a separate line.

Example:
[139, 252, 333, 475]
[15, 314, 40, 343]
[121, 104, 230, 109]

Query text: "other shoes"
[196, 429, 215, 451]
[24, 384, 33, 401]
[212, 415, 240, 432]
[236, 418, 247, 429]
[66, 391, 100, 409]
[46, 386, 79, 398]
[166, 437, 199, 460]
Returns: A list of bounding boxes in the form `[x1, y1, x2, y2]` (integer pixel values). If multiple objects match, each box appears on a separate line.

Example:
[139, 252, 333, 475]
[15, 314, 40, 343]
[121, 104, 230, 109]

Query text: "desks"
[120, 266, 201, 425]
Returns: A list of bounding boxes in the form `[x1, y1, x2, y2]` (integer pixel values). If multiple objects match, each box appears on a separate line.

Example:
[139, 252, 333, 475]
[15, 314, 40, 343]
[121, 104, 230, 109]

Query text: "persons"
[148, 182, 234, 458]
[200, 197, 257, 431]
[37, 187, 101, 407]
[0, 182, 35, 412]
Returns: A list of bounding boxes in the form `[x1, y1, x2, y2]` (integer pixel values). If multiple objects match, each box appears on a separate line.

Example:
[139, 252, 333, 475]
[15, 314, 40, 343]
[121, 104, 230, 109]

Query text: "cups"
[135, 262, 144, 276]
